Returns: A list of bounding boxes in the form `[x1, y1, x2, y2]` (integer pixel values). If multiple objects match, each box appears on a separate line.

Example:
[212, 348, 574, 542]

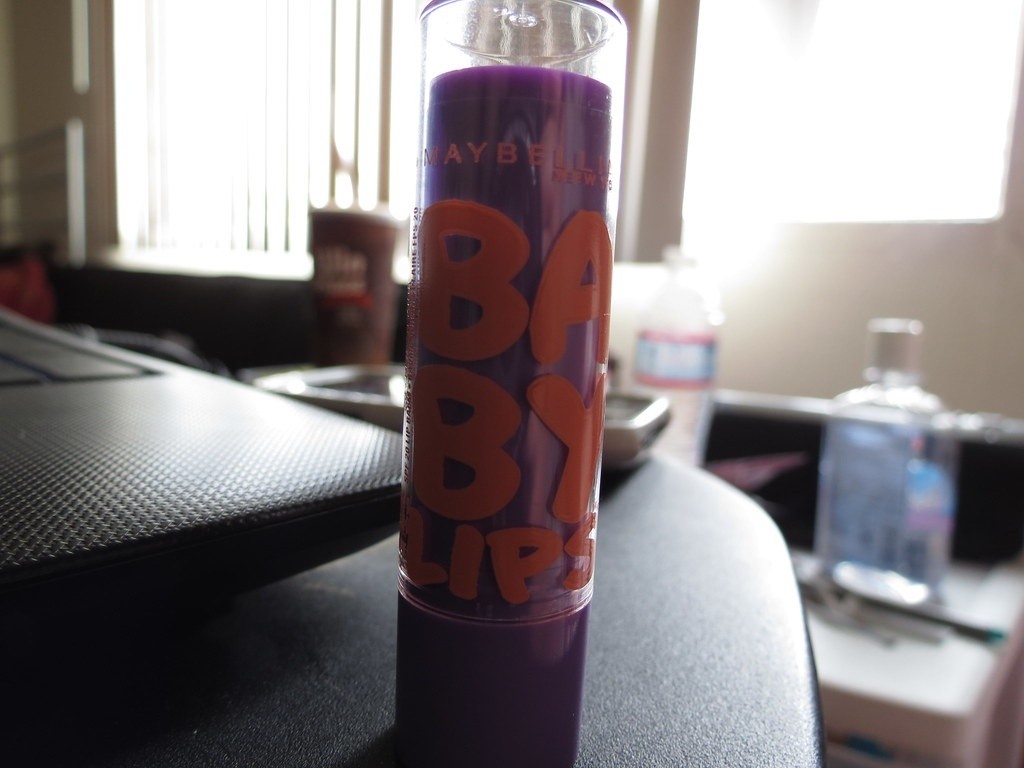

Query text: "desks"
[792, 551, 1024, 768]
[0, 449, 825, 768]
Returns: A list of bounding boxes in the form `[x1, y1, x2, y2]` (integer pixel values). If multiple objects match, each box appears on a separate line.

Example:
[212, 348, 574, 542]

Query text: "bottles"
[812, 318, 962, 639]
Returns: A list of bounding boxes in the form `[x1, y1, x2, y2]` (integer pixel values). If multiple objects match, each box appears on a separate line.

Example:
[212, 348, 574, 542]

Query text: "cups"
[394, 0, 629, 626]
